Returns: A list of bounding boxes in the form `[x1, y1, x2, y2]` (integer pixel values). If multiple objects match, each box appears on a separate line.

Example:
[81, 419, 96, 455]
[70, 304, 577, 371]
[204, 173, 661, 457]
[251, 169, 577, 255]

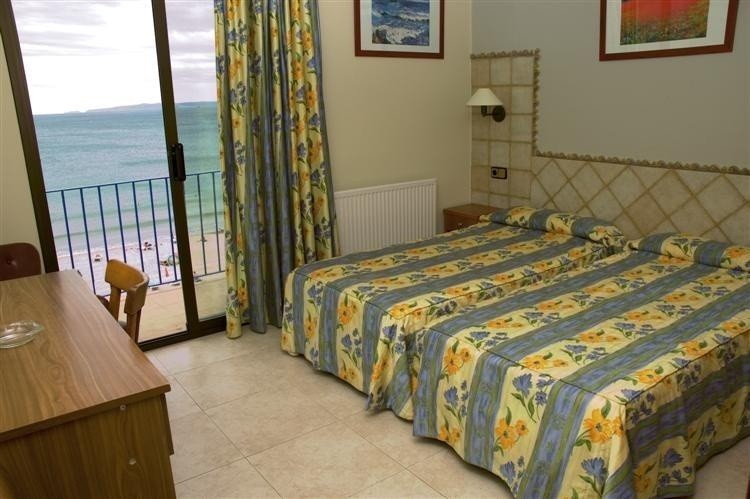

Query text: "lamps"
[467, 88, 506, 122]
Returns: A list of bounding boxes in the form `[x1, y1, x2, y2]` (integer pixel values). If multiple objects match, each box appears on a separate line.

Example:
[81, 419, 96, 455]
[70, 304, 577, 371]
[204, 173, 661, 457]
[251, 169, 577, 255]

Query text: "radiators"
[333, 178, 438, 256]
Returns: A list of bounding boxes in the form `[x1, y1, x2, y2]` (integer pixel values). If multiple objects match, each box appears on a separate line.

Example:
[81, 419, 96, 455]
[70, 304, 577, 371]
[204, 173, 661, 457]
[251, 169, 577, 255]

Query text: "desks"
[0, 270, 176, 498]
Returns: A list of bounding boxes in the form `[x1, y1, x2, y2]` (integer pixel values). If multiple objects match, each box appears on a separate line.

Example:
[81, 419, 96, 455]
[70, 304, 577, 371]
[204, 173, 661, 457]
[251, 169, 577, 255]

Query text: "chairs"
[0, 242, 41, 280]
[106, 259, 149, 342]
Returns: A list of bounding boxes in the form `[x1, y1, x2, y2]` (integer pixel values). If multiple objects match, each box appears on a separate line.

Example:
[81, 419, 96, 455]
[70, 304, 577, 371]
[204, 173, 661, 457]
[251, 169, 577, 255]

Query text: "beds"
[413, 250, 749, 499]
[290, 223, 627, 420]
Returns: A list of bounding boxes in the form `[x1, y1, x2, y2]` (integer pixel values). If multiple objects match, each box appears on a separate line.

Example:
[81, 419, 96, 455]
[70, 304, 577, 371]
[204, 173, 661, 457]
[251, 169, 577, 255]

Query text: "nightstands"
[444, 203, 498, 229]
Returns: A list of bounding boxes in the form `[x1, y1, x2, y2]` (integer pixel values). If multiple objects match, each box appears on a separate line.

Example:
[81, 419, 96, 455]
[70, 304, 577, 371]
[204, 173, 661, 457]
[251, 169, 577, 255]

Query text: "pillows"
[625, 233, 750, 272]
[479, 207, 623, 247]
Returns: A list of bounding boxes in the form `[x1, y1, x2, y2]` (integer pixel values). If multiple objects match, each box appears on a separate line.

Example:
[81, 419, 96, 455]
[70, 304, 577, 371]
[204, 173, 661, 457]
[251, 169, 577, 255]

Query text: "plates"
[0, 318, 45, 349]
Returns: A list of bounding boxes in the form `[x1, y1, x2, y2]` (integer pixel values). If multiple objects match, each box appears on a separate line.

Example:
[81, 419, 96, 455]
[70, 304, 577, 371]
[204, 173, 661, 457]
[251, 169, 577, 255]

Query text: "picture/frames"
[600, 1, 739, 60]
[355, 0, 443, 58]
